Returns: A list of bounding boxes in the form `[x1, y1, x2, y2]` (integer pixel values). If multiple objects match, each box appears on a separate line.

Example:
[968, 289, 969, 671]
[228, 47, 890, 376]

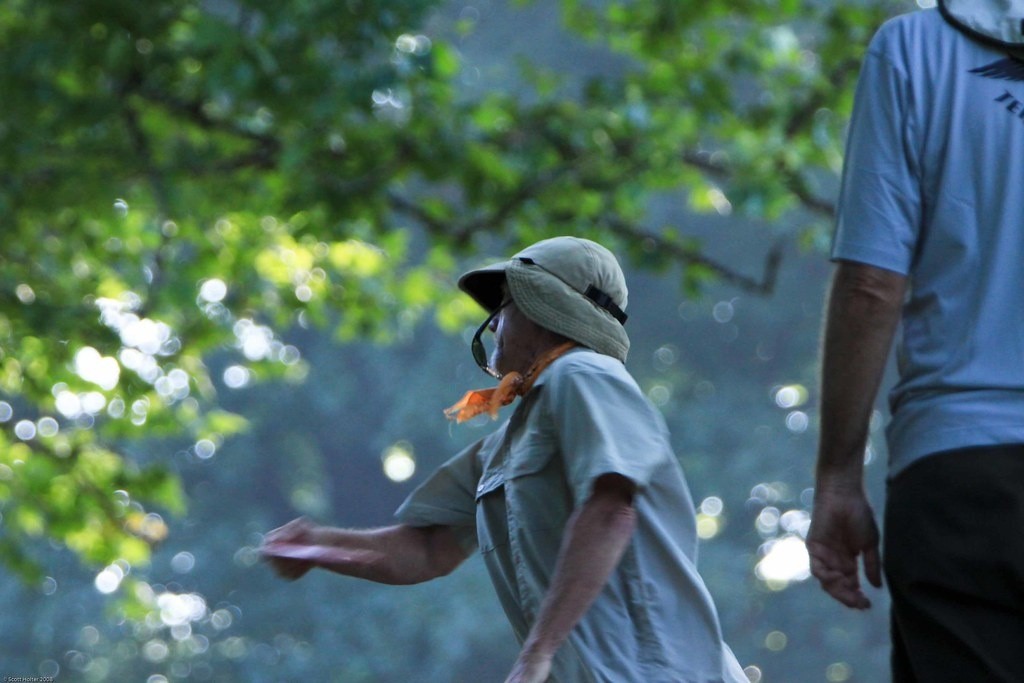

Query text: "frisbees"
[260, 541, 386, 568]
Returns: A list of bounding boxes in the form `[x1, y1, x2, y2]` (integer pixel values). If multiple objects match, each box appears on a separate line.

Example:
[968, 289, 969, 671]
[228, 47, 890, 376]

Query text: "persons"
[258, 235, 754, 683]
[802, 0, 1023, 683]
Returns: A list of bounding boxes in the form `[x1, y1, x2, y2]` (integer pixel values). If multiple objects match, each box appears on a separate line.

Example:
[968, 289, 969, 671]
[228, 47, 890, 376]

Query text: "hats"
[457, 236, 630, 361]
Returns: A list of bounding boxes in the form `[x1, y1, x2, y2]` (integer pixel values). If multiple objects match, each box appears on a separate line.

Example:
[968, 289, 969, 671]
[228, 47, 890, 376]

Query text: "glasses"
[471, 297, 514, 378]
